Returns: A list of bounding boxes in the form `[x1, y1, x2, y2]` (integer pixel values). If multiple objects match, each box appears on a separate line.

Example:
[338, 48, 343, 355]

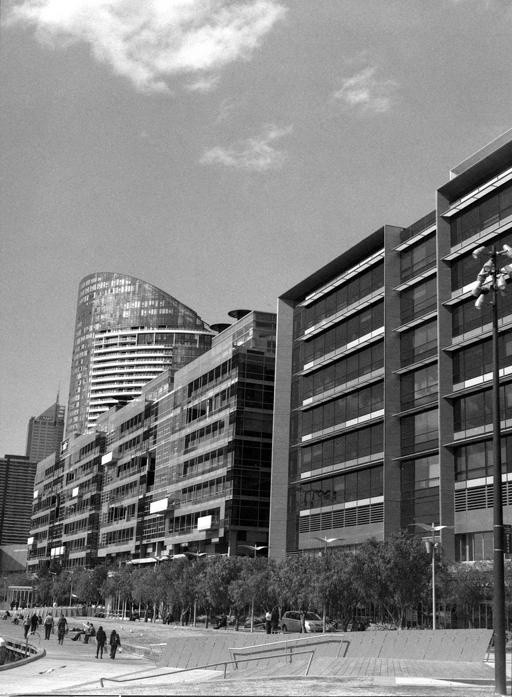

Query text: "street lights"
[468, 243, 512, 696]
[182, 550, 209, 627]
[3, 566, 100, 610]
[408, 519, 459, 632]
[150, 554, 169, 625]
[236, 542, 269, 632]
[312, 533, 347, 635]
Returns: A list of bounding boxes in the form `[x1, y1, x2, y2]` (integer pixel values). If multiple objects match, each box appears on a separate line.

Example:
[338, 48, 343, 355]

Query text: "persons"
[265, 607, 272, 633]
[213, 611, 227, 628]
[109, 629, 120, 658]
[185, 607, 192, 625]
[270, 606, 280, 633]
[95, 625, 107, 658]
[2, 597, 95, 644]
[163, 610, 173, 625]
[181, 606, 187, 625]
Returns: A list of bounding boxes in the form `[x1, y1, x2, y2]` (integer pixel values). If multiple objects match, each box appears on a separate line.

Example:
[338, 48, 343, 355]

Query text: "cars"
[188, 610, 392, 634]
